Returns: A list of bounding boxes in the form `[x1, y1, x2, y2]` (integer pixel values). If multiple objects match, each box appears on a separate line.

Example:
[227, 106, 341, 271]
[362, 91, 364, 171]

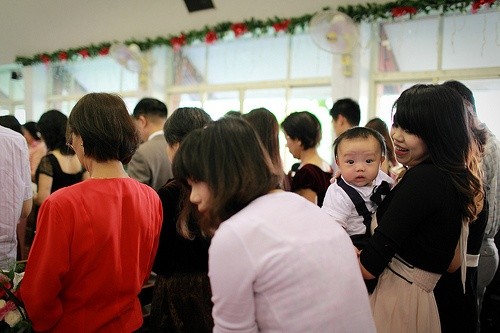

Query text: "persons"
[124, 97, 177, 193]
[34, 110, 91, 236]
[363, 116, 397, 179]
[241, 108, 291, 192]
[170, 115, 375, 333]
[328, 83, 484, 333]
[280, 111, 334, 208]
[0, 104, 52, 186]
[10, 93, 163, 333]
[438, 80, 500, 333]
[329, 99, 362, 189]
[321, 127, 398, 294]
[150, 106, 218, 333]
[0, 122, 33, 295]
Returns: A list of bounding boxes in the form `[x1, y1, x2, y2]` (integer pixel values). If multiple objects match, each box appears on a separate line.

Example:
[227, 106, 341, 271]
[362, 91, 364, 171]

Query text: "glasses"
[66, 134, 77, 150]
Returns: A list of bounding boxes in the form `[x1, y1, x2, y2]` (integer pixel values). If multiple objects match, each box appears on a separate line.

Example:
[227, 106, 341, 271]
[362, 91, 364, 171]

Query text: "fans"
[310, 9, 360, 79]
[109, 40, 150, 92]
